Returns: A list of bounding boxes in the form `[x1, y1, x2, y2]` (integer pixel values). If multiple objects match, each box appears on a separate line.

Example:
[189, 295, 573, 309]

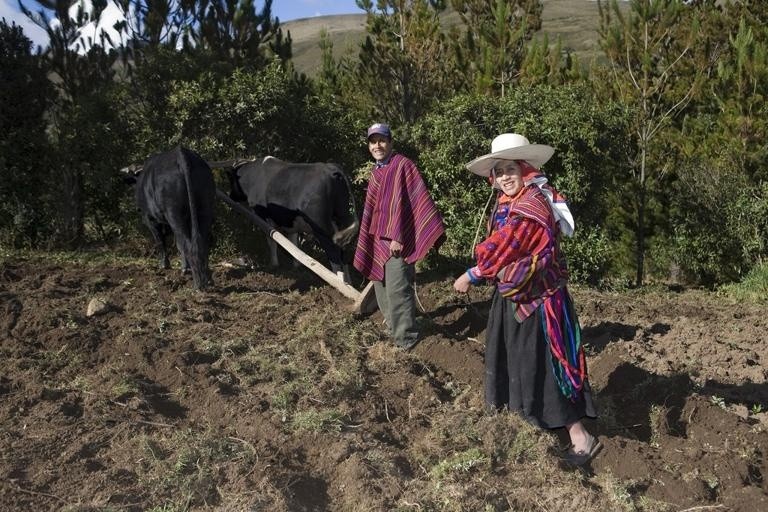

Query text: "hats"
[464, 133, 555, 178]
[367, 123, 390, 139]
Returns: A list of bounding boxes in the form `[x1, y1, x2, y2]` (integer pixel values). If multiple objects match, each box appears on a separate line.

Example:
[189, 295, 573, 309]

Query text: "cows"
[220, 156, 359, 287]
[119, 144, 215, 290]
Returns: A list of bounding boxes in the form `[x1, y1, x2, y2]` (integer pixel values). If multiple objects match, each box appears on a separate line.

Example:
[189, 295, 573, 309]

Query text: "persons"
[452, 132, 603, 472]
[352, 123, 447, 356]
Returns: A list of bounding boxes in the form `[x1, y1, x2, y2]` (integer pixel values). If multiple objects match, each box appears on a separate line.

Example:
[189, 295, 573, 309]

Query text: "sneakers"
[566, 434, 601, 466]
